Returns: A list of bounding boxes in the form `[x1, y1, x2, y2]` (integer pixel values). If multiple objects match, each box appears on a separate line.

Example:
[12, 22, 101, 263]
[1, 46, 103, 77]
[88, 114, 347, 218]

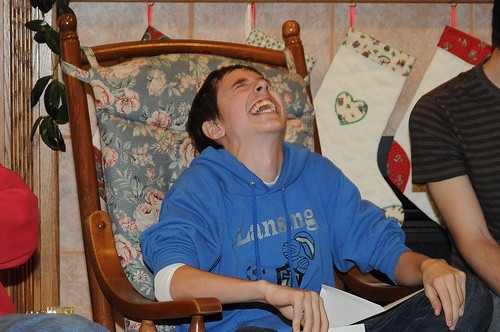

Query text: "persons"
[408, 0, 500, 332]
[0, 164, 112, 332]
[140, 64, 493, 332]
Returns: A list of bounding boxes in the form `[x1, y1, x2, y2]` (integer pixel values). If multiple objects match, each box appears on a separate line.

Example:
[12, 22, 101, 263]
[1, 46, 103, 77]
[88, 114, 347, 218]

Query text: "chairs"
[58, 13, 424, 332]
